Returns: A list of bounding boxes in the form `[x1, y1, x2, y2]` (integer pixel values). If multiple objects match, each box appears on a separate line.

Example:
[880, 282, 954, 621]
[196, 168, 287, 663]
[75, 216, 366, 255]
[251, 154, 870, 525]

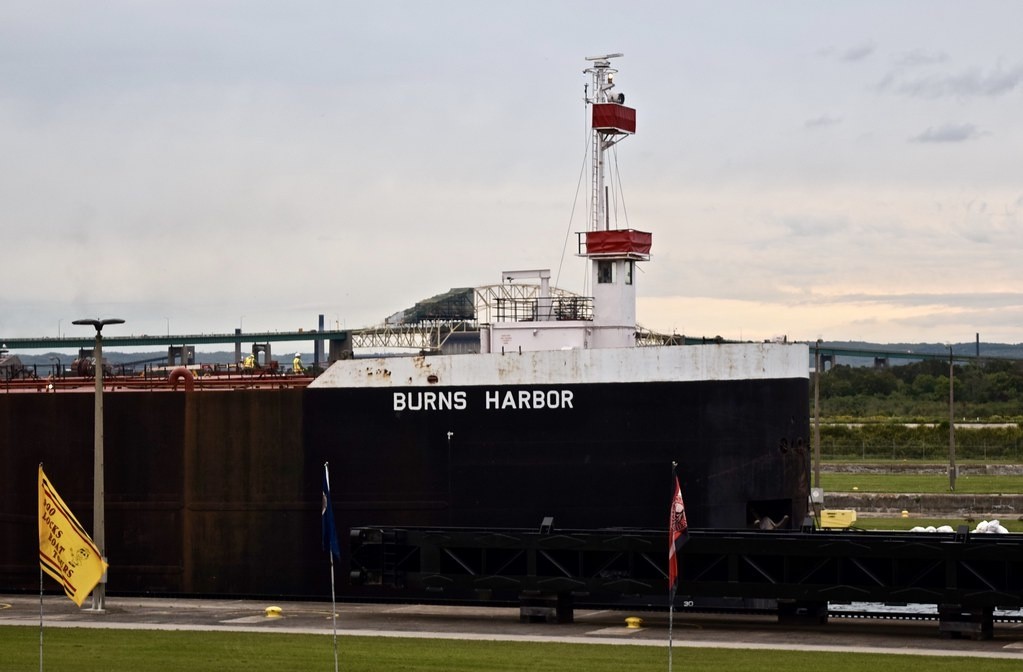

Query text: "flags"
[36, 464, 106, 609]
[320, 466, 341, 563]
[667, 448, 691, 591]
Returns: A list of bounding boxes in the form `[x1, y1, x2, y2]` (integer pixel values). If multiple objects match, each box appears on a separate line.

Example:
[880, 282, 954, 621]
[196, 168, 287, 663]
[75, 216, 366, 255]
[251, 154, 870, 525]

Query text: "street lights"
[58, 318, 64, 340]
[240, 314, 246, 336]
[162, 316, 170, 338]
[71, 319, 126, 613]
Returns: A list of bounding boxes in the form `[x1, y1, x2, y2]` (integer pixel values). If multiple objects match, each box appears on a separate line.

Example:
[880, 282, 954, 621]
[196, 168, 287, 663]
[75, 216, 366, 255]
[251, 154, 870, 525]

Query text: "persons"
[293, 352, 306, 375]
[244, 354, 255, 374]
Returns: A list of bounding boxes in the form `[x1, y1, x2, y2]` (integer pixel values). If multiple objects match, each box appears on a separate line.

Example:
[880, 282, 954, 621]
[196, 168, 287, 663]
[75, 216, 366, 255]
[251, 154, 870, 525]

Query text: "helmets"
[251, 354, 254, 358]
[295, 353, 300, 356]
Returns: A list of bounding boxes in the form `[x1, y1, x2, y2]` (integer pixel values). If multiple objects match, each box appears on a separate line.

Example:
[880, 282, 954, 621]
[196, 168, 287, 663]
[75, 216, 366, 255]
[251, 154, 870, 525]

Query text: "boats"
[0, 52, 813, 612]
